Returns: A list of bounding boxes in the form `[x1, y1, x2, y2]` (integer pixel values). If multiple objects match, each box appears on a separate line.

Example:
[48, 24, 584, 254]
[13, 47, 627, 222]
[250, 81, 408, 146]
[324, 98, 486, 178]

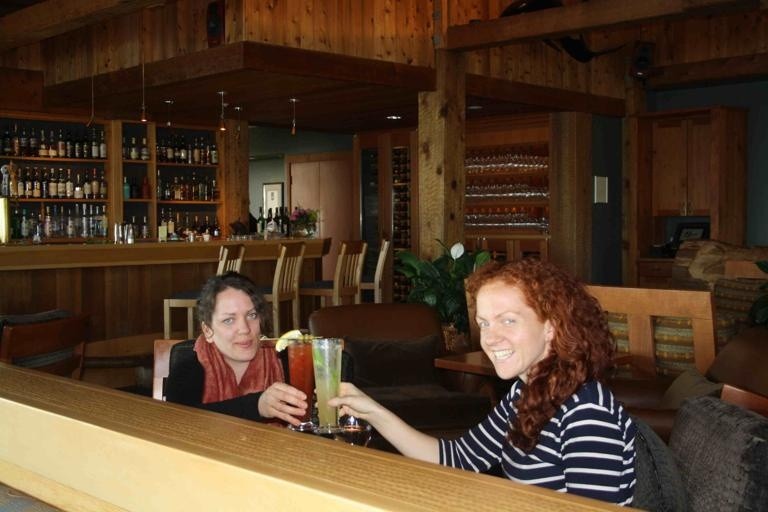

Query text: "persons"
[165, 272, 308, 427]
[705, 295, 768, 397]
[314, 259, 636, 508]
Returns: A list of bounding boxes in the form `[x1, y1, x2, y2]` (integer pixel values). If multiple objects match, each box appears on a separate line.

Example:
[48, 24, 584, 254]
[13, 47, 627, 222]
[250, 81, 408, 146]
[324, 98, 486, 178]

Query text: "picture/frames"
[262, 182, 283, 221]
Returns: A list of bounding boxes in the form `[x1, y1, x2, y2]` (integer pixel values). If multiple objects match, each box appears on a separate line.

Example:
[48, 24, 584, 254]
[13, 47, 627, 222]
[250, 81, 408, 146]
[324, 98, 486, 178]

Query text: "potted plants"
[393, 240, 491, 353]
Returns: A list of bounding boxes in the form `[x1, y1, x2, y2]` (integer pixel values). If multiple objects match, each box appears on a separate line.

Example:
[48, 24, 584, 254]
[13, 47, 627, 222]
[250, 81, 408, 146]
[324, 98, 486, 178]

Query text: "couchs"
[462, 278, 768, 381]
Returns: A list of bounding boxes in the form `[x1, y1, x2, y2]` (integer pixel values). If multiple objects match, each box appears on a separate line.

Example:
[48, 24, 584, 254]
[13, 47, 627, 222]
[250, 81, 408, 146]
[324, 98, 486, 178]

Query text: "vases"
[290, 225, 316, 240]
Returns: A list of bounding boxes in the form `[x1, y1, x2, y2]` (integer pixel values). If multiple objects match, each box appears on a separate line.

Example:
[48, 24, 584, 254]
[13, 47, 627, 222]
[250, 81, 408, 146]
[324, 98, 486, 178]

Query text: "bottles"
[1, 123, 222, 246]
[255, 206, 291, 239]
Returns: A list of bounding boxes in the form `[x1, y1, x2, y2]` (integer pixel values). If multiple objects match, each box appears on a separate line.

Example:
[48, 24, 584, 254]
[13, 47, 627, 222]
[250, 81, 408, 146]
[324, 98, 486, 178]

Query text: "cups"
[307, 338, 344, 434]
[285, 337, 316, 432]
[339, 414, 373, 448]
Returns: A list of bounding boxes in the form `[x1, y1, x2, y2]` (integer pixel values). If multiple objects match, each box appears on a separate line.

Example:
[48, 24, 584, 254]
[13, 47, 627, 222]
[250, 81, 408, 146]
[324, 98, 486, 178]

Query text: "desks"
[78, 331, 186, 370]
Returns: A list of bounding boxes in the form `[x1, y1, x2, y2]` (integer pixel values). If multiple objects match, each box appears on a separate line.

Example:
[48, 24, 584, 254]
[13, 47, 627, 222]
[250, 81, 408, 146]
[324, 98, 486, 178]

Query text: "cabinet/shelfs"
[353, 127, 418, 303]
[652, 114, 748, 216]
[639, 256, 673, 289]
[0, 109, 222, 243]
[465, 172, 549, 266]
[285, 155, 353, 306]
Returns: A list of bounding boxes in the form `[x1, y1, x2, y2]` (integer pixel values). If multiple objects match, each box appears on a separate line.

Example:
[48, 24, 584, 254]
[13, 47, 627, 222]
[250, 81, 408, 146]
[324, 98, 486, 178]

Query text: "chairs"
[299, 240, 367, 305]
[0, 309, 91, 380]
[153, 337, 287, 403]
[264, 242, 306, 337]
[310, 303, 487, 430]
[164, 299, 198, 339]
[164, 244, 246, 339]
[360, 238, 390, 303]
[669, 384, 768, 512]
[613, 324, 768, 443]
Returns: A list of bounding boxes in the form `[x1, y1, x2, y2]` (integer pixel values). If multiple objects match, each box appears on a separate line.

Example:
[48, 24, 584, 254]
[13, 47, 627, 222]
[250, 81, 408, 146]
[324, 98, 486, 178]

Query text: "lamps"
[140, 53, 148, 122]
[219, 92, 225, 131]
[86, 76, 94, 128]
[290, 99, 296, 135]
[235, 107, 241, 139]
[165, 100, 172, 127]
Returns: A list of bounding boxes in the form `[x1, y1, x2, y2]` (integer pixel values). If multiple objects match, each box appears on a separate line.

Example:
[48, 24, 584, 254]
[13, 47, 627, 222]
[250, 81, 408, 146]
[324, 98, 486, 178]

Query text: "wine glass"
[463, 154, 548, 236]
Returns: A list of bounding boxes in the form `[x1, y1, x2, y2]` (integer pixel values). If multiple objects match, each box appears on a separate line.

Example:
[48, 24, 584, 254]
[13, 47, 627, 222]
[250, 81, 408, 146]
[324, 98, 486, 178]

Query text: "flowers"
[287, 209, 318, 231]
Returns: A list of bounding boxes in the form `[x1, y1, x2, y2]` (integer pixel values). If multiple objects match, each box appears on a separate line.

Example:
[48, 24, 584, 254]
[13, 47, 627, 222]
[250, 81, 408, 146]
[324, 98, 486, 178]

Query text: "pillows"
[659, 368, 723, 411]
[342, 334, 438, 387]
